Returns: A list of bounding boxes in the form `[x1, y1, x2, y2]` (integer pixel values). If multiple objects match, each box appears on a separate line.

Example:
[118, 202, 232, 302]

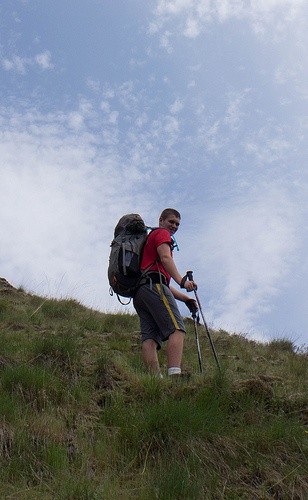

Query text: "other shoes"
[167, 370, 192, 378]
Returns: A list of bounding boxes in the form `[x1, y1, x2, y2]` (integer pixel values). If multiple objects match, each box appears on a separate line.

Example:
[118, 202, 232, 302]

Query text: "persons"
[133, 208, 199, 379]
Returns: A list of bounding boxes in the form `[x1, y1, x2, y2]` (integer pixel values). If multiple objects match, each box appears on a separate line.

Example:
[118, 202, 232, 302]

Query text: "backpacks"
[108, 213, 163, 298]
